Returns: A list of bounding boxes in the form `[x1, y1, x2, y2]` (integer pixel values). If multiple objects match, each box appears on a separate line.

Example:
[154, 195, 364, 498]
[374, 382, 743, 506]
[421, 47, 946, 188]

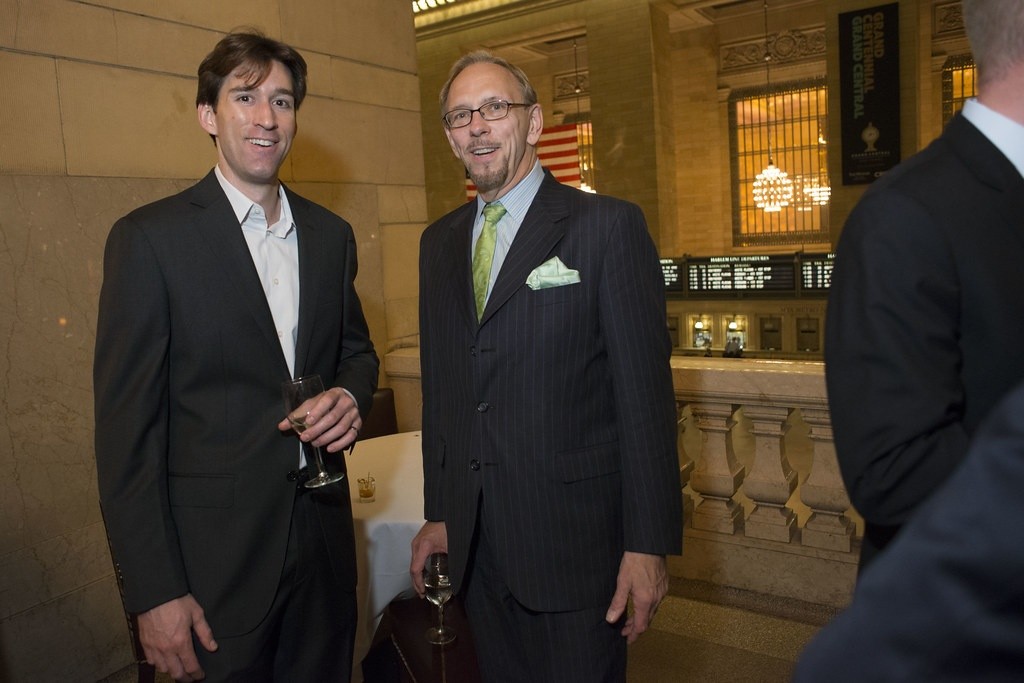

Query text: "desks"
[344, 430, 427, 667]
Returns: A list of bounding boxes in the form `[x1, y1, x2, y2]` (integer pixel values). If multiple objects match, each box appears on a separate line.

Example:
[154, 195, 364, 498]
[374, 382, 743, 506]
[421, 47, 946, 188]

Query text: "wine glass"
[422, 553, 457, 644]
[281, 374, 345, 489]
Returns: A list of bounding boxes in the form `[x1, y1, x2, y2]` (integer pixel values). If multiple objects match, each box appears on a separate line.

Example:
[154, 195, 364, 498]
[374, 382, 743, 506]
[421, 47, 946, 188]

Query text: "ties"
[472, 204, 507, 323]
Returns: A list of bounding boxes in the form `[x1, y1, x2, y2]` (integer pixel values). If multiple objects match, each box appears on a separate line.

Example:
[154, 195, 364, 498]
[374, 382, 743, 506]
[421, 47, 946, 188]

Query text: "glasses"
[441, 100, 533, 130]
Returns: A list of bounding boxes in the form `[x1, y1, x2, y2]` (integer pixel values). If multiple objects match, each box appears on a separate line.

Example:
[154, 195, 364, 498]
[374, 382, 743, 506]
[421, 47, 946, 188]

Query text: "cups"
[356, 477, 377, 503]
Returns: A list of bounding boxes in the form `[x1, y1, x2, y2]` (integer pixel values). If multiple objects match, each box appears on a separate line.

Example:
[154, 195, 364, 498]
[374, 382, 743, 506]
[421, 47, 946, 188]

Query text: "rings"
[348, 427, 360, 436]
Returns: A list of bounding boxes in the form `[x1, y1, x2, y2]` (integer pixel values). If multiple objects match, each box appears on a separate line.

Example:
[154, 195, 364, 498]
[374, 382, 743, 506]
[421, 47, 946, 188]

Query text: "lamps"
[572, 36, 596, 194]
[753, 0, 793, 213]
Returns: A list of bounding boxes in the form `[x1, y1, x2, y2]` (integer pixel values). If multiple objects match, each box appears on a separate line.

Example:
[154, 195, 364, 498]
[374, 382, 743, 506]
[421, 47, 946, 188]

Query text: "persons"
[798, 0, 1024, 683]
[408, 51, 687, 683]
[94, 24, 379, 683]
